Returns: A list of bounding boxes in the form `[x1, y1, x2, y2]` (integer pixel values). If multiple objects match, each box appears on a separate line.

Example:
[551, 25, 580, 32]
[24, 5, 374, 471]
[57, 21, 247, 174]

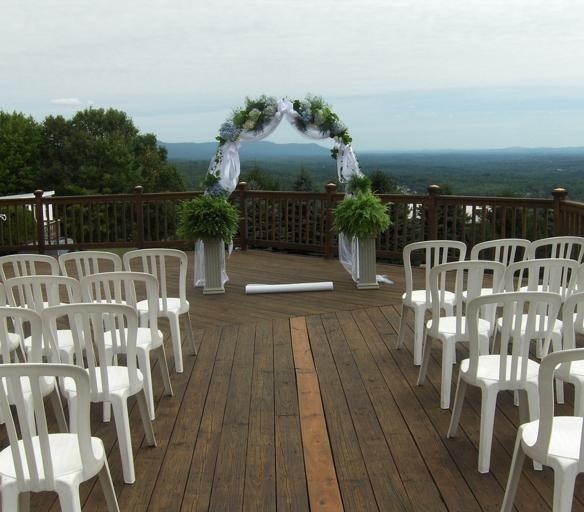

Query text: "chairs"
[0, 364, 120, 512]
[124, 249, 196, 374]
[445, 292, 560, 474]
[2, 253, 69, 327]
[2, 275, 92, 369]
[418, 262, 507, 410]
[554, 290, 582, 417]
[80, 271, 173, 421]
[396, 241, 467, 367]
[58, 251, 127, 321]
[1, 307, 71, 436]
[472, 237, 582, 403]
[40, 302, 157, 485]
[500, 349, 584, 511]
[2, 306, 46, 371]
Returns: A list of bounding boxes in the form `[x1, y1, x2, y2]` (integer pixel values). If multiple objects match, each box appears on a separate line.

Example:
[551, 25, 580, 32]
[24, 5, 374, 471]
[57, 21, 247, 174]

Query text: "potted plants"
[174, 197, 240, 295]
[328, 189, 391, 291]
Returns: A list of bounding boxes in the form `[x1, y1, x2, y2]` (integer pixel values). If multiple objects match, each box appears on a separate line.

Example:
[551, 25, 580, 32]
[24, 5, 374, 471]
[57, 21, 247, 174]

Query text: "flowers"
[211, 89, 353, 162]
